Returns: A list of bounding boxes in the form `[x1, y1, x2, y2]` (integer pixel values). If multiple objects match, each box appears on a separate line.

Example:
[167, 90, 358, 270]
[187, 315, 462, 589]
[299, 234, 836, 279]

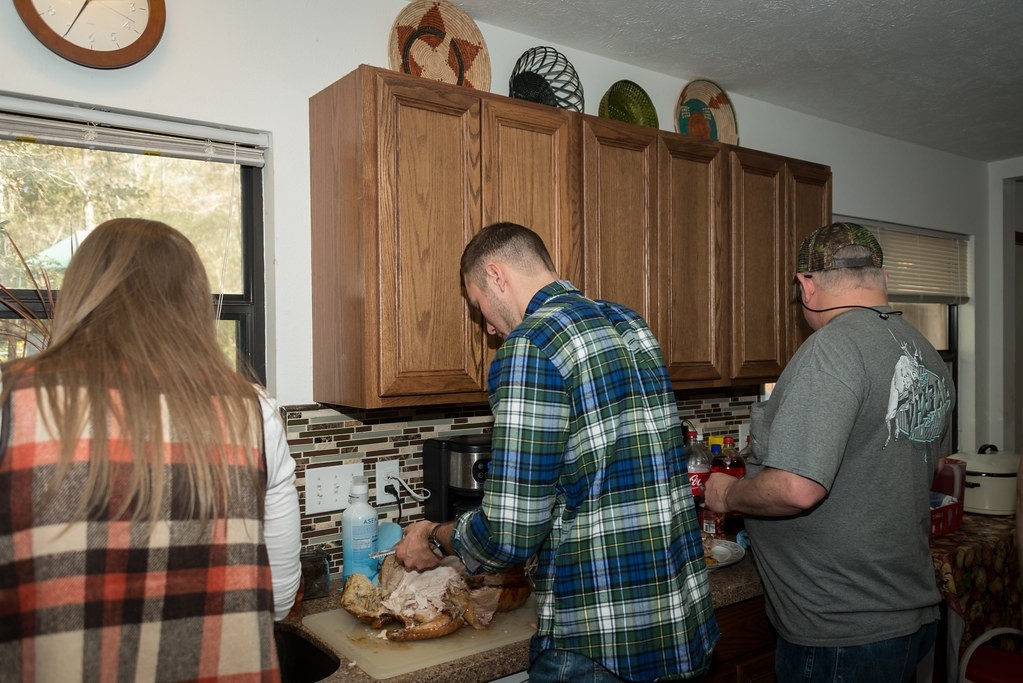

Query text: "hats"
[795, 221, 885, 272]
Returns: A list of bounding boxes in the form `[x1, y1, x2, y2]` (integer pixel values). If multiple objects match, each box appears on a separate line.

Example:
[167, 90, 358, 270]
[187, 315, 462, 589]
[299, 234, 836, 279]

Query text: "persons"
[705, 222, 956, 683]
[392, 222, 721, 683]
[1, 217, 302, 683]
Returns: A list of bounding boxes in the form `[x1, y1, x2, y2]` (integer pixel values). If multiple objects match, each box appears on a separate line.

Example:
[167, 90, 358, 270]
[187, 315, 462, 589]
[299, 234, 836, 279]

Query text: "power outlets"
[376, 459, 401, 505]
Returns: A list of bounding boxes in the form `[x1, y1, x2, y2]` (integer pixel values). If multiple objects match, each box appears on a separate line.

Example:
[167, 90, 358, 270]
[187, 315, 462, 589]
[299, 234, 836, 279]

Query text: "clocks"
[12, 0, 168, 70]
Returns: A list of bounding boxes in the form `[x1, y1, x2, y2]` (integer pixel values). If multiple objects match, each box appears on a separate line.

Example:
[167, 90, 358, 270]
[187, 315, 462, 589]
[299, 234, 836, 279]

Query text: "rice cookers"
[948, 440, 1023, 516]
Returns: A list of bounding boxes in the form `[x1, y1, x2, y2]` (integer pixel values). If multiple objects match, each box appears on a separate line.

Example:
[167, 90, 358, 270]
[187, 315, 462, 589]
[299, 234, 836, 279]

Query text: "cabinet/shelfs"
[707, 595, 782, 682]
[581, 113, 731, 398]
[309, 64, 580, 413]
[733, 147, 832, 384]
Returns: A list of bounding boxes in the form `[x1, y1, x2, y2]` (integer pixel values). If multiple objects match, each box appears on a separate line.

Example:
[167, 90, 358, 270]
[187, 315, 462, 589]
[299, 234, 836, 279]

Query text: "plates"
[700, 538, 745, 572]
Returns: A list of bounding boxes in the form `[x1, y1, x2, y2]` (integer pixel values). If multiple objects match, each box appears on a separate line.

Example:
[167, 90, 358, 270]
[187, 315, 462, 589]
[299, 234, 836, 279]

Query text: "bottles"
[342, 473, 379, 590]
[685, 428, 748, 543]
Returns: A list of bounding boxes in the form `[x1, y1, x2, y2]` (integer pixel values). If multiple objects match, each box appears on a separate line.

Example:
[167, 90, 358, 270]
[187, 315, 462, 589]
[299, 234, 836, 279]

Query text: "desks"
[917, 511, 1021, 683]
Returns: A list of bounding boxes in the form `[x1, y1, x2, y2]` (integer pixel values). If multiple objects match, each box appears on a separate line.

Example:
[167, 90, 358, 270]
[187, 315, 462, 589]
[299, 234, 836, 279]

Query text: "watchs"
[428, 525, 448, 559]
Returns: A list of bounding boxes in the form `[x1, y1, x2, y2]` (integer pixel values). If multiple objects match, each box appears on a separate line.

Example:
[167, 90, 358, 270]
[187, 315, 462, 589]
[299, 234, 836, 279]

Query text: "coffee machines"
[434, 428, 496, 527]
